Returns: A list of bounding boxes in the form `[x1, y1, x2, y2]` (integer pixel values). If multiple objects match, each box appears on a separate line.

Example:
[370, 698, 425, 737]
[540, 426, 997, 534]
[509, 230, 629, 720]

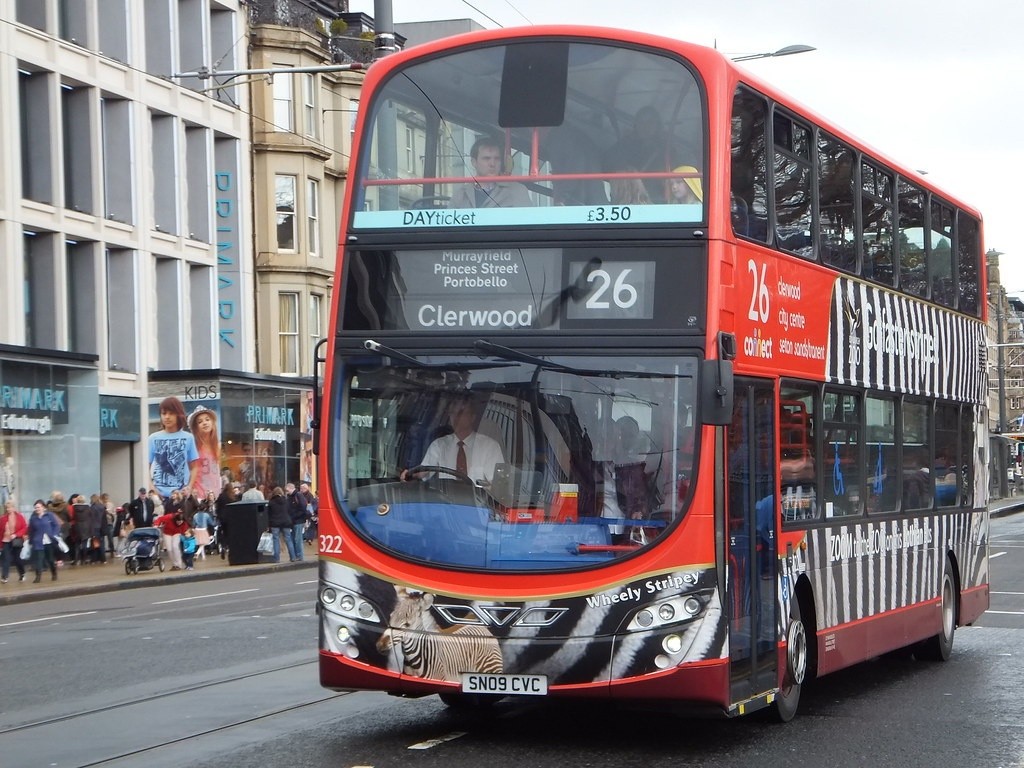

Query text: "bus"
[296, 29, 992, 731]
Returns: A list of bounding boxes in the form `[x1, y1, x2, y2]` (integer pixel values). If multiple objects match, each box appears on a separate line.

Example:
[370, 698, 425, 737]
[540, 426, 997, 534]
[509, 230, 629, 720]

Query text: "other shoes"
[18, 574, 25, 581]
[1, 576, 8, 583]
[169, 566, 180, 571]
[51, 572, 57, 580]
[184, 566, 194, 570]
[33, 575, 41, 583]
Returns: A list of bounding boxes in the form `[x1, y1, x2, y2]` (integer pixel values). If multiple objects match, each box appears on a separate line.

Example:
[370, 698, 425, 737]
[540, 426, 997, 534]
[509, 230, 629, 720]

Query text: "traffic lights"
[1019, 443, 1024, 452]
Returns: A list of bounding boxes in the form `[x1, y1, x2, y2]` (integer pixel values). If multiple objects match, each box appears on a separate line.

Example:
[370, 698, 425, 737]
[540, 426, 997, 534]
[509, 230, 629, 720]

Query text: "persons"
[0, 500, 29, 583]
[399, 393, 505, 509]
[28, 500, 61, 583]
[45, 491, 116, 568]
[537, 100, 702, 204]
[221, 467, 231, 486]
[0, 456, 17, 508]
[186, 404, 220, 505]
[590, 401, 694, 547]
[149, 396, 199, 506]
[215, 480, 319, 562]
[303, 399, 314, 474]
[112, 487, 218, 572]
[445, 137, 534, 207]
[238, 444, 263, 485]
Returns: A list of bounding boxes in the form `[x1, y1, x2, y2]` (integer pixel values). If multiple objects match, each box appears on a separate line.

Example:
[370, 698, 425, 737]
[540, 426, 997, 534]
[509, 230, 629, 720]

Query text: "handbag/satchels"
[295, 497, 314, 515]
[54, 535, 69, 553]
[20, 540, 32, 559]
[256, 529, 275, 556]
[12, 537, 23, 547]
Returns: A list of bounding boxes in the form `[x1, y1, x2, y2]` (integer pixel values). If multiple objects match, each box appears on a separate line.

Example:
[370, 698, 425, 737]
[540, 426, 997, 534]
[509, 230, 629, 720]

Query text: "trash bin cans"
[225, 499, 274, 565]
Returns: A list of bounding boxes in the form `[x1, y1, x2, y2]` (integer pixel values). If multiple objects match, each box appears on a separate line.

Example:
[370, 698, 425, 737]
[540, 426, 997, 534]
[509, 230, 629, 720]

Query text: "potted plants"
[329, 18, 347, 64]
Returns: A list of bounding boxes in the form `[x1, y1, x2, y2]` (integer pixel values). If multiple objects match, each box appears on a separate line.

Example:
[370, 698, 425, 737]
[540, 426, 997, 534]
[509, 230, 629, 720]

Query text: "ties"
[456, 440, 467, 480]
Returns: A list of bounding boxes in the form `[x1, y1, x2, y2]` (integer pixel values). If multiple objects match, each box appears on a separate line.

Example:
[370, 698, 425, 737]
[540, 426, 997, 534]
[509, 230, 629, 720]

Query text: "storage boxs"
[507, 483, 579, 524]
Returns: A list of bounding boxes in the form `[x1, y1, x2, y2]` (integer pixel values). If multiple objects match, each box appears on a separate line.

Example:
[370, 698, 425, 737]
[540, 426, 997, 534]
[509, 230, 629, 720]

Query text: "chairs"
[734, 197, 977, 315]
[477, 417, 508, 466]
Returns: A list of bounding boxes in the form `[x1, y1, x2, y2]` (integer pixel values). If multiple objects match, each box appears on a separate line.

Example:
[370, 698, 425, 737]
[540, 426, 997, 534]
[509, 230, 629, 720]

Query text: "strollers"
[125, 524, 165, 574]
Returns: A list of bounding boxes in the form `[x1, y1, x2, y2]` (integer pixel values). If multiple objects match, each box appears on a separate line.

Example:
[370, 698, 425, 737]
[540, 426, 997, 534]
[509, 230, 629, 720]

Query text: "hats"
[186, 405, 216, 431]
[139, 487, 146, 494]
